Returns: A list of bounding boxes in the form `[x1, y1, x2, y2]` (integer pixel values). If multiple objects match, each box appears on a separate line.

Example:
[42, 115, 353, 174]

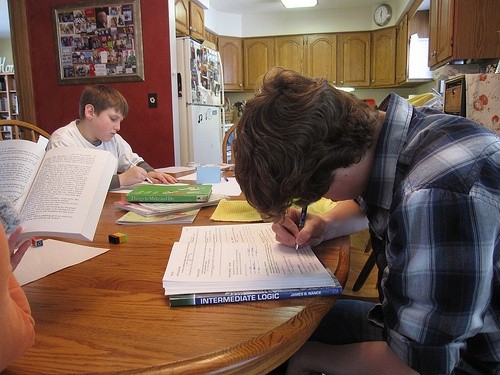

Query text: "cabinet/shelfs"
[0, 73, 20, 141]
[175, 0, 206, 42]
[203, 0, 500, 90]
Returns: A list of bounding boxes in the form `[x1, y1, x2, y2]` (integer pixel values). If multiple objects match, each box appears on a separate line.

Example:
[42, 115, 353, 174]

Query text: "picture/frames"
[51, 0, 145, 85]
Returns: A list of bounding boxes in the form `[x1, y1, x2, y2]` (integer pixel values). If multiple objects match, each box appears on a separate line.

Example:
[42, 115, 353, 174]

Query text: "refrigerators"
[176, 36, 225, 167]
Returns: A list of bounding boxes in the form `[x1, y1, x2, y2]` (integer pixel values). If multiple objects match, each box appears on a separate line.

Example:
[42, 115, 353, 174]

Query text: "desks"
[0, 167, 351, 375]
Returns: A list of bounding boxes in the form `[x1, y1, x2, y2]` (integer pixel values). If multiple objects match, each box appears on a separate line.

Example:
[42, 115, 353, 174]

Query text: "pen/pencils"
[125, 156, 153, 184]
[295, 206, 308, 251]
[224, 176, 228, 182]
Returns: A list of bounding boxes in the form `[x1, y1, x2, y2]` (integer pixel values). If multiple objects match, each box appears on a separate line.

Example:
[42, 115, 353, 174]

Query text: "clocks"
[373, 4, 392, 27]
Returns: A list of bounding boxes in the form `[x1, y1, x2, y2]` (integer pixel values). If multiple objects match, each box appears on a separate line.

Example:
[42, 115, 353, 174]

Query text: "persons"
[59, 5, 135, 78]
[235, 66, 500, 375]
[45, 85, 179, 190]
[0, 223, 36, 375]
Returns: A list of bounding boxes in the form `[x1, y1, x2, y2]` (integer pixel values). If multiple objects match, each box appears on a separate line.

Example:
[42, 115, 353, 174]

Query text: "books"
[210, 193, 338, 223]
[0, 141, 118, 243]
[107, 162, 242, 195]
[115, 196, 225, 216]
[115, 208, 202, 224]
[170, 258, 343, 306]
[164, 223, 336, 296]
[126, 184, 212, 202]
